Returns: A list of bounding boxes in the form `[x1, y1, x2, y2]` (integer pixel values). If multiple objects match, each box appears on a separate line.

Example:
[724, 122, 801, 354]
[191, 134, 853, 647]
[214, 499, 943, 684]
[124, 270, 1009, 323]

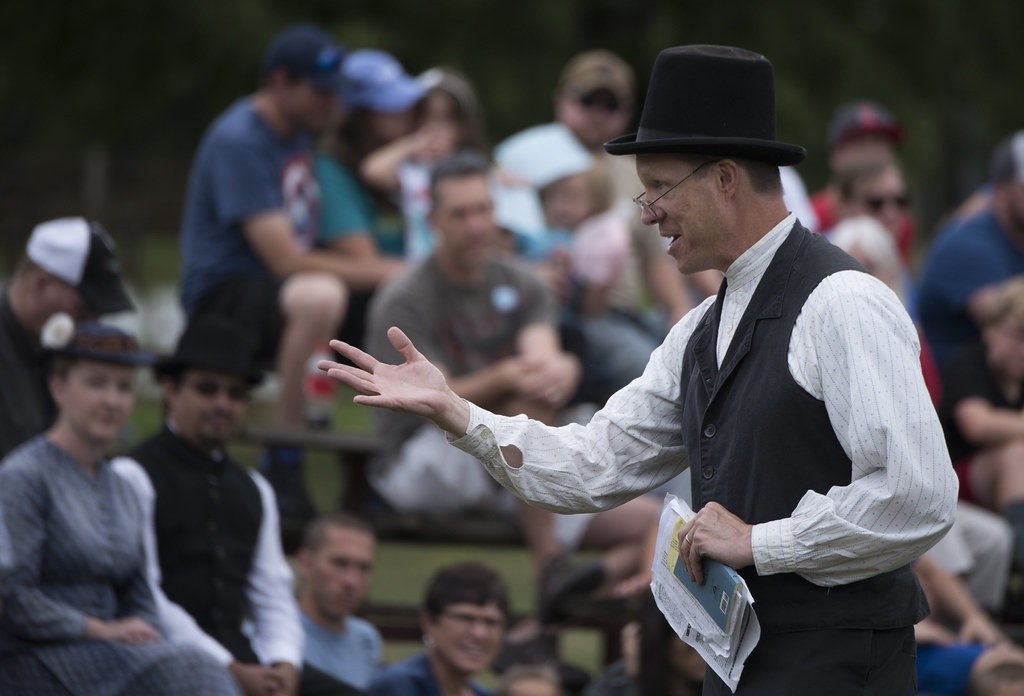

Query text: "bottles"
[301, 340, 337, 435]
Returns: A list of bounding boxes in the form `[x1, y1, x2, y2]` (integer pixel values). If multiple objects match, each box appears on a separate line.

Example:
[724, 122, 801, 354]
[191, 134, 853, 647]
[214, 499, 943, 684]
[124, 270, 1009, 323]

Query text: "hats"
[557, 50, 633, 113]
[823, 99, 902, 156]
[416, 66, 476, 125]
[148, 320, 260, 392]
[38, 312, 157, 369]
[986, 132, 1024, 181]
[24, 215, 135, 313]
[339, 47, 423, 117]
[271, 20, 346, 92]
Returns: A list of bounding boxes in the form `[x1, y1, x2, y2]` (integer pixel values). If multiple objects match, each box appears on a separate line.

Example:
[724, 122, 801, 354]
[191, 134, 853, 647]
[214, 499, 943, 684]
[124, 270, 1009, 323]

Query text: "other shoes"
[533, 557, 605, 623]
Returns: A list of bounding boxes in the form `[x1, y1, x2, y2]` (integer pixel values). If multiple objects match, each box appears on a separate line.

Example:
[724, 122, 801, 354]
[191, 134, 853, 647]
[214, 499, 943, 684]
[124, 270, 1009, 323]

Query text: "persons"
[2, 324, 239, 696]
[0, 21, 1024, 696]
[322, 44, 961, 695]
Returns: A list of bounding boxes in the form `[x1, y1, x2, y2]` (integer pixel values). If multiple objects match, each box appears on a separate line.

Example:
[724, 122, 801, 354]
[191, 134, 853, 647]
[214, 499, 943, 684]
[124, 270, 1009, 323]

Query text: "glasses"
[631, 157, 723, 218]
[177, 378, 252, 408]
[848, 193, 910, 214]
[434, 608, 503, 639]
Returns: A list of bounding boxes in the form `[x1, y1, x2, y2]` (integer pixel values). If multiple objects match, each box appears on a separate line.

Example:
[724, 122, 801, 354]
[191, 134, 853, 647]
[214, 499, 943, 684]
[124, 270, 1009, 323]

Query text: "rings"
[686, 534, 691, 545]
